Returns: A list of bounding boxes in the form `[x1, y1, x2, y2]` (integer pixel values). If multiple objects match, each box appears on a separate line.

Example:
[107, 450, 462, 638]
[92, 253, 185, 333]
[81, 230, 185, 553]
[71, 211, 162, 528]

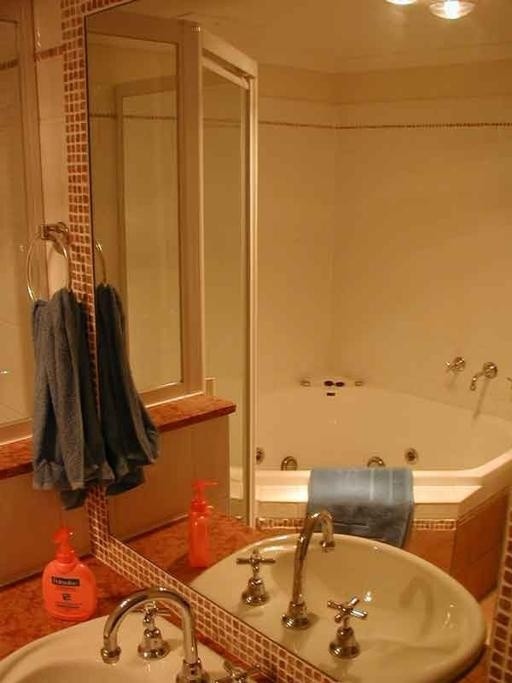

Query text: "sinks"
[0, 614, 257, 683]
[189, 534, 489, 683]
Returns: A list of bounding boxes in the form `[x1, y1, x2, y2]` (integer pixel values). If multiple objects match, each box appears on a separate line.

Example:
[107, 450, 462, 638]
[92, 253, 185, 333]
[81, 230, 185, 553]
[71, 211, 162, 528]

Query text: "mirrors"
[58, 0, 512, 683]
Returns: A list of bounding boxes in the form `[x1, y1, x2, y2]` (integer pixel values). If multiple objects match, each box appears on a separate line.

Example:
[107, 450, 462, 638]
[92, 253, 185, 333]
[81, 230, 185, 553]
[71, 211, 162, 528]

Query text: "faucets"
[468, 364, 497, 389]
[280, 511, 336, 627]
[102, 585, 211, 682]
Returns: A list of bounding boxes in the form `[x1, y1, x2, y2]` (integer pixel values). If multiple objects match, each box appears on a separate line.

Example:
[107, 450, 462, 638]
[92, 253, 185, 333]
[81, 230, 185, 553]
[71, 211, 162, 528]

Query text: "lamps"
[429, 0, 474, 20]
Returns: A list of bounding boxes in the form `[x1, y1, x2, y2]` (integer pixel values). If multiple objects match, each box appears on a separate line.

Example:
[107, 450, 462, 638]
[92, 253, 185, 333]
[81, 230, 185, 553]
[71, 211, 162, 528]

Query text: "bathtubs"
[223, 381, 512, 482]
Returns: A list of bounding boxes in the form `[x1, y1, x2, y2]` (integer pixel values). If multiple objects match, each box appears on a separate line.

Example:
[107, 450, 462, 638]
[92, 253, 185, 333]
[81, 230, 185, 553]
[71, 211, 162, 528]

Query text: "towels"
[29, 289, 95, 511]
[95, 281, 159, 483]
[307, 467, 411, 549]
